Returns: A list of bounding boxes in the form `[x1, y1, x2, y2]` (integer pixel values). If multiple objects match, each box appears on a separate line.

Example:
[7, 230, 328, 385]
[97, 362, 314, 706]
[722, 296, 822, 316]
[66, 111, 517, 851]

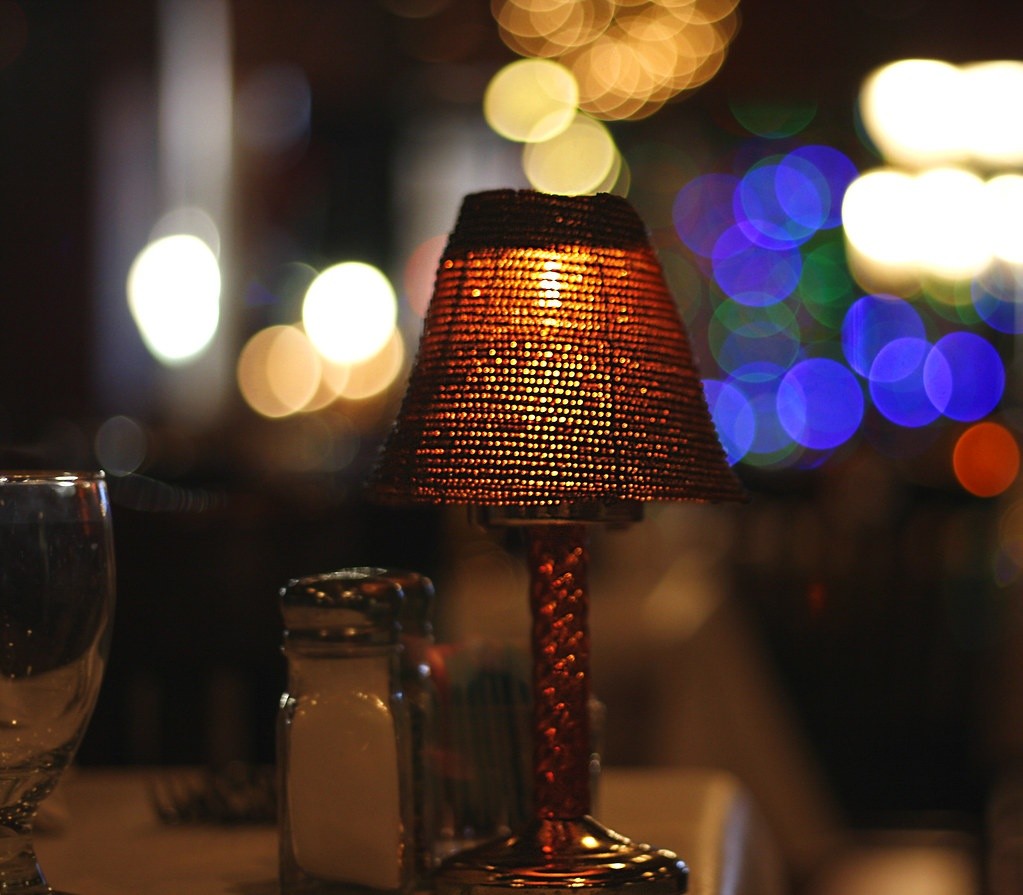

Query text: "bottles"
[380, 571, 434, 650]
[276, 572, 407, 892]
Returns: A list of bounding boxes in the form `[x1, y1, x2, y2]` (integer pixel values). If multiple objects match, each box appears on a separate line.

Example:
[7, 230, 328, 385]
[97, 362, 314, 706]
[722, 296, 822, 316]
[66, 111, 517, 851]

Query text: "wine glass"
[0, 467, 115, 895]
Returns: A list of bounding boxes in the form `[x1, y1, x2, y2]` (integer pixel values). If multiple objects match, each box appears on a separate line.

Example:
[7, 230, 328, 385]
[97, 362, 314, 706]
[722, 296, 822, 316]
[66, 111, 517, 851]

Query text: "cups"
[410, 642, 533, 886]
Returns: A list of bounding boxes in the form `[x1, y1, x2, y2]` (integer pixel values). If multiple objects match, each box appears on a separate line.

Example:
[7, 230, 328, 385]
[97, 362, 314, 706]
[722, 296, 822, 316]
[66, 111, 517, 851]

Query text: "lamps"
[367, 188, 752, 895]
